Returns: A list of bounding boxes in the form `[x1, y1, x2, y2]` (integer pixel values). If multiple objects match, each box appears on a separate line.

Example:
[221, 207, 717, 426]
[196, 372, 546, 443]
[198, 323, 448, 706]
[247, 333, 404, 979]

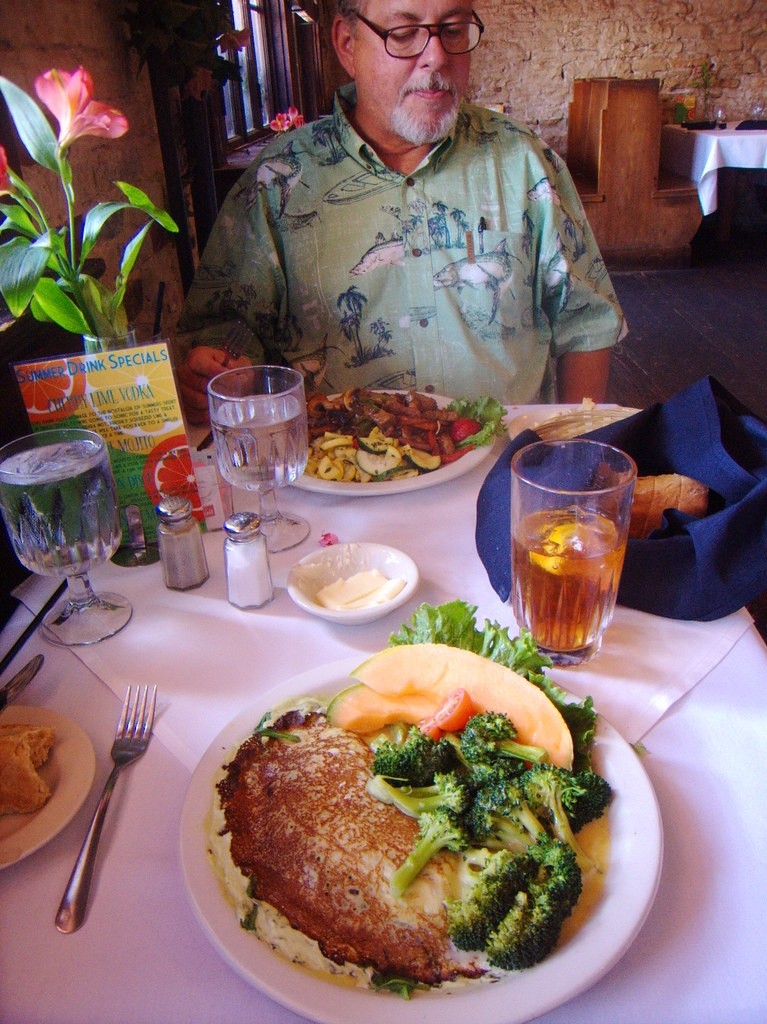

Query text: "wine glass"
[0, 430, 134, 645]
[208, 366, 311, 553]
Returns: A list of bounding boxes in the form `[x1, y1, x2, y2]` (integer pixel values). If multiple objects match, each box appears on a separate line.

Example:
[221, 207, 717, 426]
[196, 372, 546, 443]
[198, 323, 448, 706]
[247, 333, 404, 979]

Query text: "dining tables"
[0, 402, 767, 1023]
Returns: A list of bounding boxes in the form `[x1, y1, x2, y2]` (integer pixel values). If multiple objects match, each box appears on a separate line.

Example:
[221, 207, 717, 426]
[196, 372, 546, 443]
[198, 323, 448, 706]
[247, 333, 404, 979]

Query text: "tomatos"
[418, 718, 440, 740]
[435, 689, 473, 732]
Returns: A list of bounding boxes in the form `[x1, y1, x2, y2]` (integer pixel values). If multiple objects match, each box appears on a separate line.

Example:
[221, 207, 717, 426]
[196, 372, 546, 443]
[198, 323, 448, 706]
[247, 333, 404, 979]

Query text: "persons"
[175, 0, 630, 427]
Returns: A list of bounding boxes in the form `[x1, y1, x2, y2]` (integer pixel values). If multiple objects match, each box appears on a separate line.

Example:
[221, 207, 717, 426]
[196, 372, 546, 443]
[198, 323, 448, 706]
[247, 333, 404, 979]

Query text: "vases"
[694, 96, 709, 122]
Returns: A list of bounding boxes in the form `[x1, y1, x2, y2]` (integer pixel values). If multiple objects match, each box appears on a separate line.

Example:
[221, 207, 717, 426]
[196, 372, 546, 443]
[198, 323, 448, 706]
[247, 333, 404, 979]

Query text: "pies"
[207, 697, 489, 989]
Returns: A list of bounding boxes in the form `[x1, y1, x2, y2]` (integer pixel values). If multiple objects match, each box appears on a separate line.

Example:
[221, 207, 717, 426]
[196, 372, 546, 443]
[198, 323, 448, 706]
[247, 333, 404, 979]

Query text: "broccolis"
[367, 710, 611, 969]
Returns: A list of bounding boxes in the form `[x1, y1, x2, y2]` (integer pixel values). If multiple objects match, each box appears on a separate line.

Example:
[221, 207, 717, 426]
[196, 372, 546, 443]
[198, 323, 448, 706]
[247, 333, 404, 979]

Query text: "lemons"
[527, 524, 583, 575]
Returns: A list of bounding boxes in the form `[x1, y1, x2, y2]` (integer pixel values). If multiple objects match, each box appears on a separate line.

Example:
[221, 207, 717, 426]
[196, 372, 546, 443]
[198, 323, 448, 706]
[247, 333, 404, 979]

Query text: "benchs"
[563, 77, 702, 267]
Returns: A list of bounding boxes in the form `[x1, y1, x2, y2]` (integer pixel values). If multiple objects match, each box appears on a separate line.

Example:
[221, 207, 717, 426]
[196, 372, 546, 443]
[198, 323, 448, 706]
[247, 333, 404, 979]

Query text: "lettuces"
[446, 392, 508, 447]
[389, 599, 596, 772]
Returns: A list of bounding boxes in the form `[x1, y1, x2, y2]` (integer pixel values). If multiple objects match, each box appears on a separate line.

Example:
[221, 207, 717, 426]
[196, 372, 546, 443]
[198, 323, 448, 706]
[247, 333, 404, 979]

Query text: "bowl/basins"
[287, 543, 419, 625]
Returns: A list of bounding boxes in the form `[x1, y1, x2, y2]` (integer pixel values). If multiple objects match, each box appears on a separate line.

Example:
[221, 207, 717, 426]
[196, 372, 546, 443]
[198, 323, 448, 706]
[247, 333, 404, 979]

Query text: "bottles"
[222, 512, 274, 610]
[156, 496, 210, 592]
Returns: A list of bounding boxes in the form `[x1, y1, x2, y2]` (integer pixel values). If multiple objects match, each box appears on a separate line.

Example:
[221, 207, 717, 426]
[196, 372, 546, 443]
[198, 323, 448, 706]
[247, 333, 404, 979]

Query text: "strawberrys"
[450, 417, 481, 442]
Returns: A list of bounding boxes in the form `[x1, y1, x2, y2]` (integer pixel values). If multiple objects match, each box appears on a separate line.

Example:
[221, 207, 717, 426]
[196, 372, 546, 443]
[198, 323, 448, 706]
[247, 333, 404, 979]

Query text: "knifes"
[0, 654, 45, 714]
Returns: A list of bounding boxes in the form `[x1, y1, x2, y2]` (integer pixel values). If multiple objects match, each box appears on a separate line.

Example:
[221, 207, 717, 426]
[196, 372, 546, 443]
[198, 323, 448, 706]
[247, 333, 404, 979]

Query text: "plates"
[179, 659, 665, 1024]
[0, 707, 96, 869]
[288, 388, 498, 497]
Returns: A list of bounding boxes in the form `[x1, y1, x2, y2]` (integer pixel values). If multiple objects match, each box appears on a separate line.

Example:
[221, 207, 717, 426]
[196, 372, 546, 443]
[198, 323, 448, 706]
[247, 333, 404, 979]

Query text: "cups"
[510, 438, 637, 669]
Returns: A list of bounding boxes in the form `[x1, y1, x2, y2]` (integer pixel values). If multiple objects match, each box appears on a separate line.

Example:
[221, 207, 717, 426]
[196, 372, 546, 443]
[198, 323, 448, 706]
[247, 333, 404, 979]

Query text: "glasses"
[352, 8, 485, 58]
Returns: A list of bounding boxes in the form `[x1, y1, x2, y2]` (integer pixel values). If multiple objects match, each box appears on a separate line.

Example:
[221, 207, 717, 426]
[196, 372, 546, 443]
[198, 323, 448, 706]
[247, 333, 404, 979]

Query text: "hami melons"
[326, 643, 575, 771]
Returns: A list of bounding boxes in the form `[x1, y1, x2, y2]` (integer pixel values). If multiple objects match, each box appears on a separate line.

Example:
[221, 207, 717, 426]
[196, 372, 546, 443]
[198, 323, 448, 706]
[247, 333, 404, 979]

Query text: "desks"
[659, 120, 767, 241]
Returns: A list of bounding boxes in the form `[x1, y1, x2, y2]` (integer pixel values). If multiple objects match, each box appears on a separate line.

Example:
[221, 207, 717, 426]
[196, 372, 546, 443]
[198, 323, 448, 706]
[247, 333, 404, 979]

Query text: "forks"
[54, 685, 159, 935]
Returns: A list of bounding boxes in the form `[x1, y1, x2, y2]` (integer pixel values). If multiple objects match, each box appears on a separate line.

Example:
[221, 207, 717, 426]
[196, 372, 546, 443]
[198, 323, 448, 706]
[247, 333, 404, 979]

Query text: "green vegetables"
[370, 973, 432, 999]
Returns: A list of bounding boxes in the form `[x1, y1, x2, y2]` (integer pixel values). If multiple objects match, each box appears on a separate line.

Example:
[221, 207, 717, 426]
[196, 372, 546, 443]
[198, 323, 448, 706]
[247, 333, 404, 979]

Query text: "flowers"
[0, 64, 179, 339]
[118, 0, 251, 102]
[270, 107, 308, 137]
[688, 63, 719, 93]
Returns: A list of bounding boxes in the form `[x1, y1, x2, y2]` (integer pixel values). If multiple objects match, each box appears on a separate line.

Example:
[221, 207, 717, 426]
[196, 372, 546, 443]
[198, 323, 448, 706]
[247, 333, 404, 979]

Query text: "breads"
[0, 722, 57, 815]
[605, 473, 712, 539]
